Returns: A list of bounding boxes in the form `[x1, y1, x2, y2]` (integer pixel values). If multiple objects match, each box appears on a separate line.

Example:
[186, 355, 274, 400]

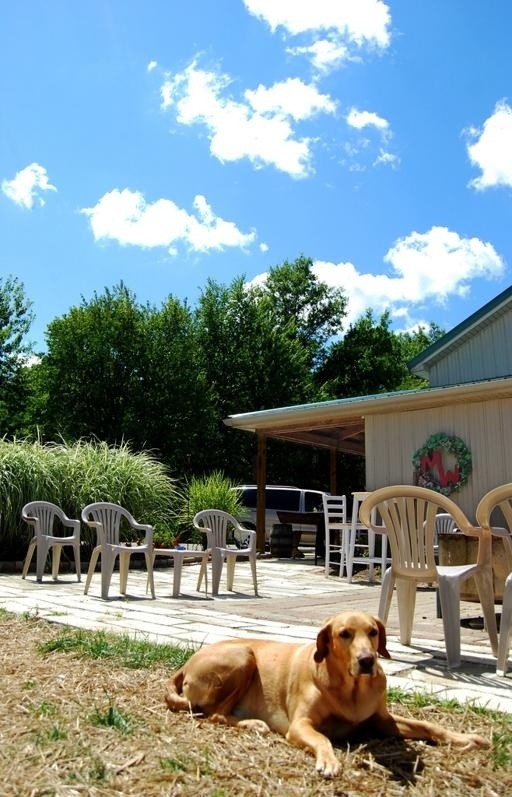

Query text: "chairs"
[358, 485, 500, 671]
[21, 500, 81, 585]
[346, 492, 391, 585]
[192, 510, 259, 596]
[84, 501, 155, 598]
[475, 480, 511, 678]
[323, 494, 368, 578]
[419, 511, 458, 558]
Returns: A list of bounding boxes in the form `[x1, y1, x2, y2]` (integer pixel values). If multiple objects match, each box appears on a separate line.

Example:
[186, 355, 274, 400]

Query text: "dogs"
[163, 609, 493, 781]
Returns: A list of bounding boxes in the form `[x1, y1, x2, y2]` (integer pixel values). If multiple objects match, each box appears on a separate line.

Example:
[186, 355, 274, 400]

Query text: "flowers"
[412, 432, 473, 498]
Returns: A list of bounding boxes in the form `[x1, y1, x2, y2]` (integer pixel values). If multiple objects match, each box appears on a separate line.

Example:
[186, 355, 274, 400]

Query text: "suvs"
[226, 483, 332, 549]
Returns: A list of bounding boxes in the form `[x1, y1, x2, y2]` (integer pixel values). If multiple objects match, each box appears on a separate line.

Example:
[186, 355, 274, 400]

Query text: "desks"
[434, 533, 509, 619]
[146, 547, 207, 597]
[276, 511, 326, 564]
